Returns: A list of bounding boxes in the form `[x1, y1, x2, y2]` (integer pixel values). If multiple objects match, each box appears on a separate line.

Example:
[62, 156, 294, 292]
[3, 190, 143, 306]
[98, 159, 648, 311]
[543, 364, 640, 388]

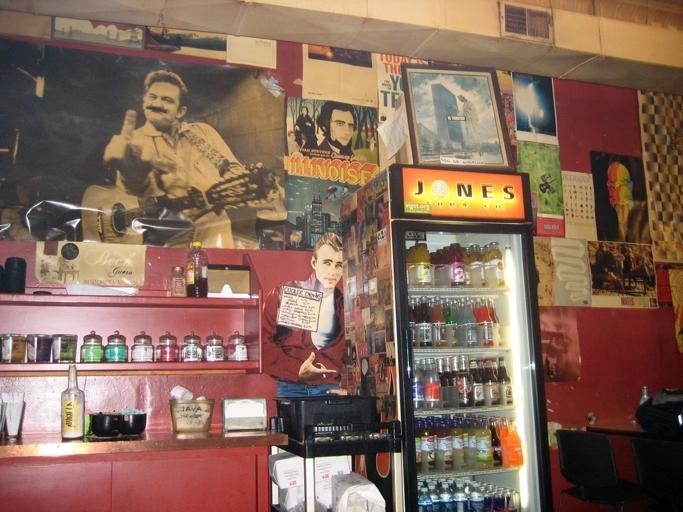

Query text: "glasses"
[605, 180, 624, 188]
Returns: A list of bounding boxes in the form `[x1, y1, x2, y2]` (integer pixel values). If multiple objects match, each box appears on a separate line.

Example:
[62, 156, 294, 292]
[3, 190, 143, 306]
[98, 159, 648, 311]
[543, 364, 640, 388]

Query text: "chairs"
[629, 436, 683, 512]
[555, 429, 641, 512]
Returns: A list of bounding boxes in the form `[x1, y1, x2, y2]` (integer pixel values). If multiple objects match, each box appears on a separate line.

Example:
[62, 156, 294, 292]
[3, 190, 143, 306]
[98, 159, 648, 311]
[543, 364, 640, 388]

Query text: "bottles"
[0, 330, 249, 363]
[171, 240, 208, 298]
[640, 385, 649, 405]
[61, 366, 85, 440]
[406, 241, 520, 511]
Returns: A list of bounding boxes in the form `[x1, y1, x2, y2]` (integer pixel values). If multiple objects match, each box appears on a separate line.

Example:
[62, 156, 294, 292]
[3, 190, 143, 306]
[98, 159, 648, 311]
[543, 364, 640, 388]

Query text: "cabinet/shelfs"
[1, 240, 288, 512]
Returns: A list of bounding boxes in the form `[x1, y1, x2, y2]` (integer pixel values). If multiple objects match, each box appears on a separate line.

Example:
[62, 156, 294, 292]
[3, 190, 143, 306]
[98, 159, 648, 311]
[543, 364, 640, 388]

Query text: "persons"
[588, 163, 655, 295]
[103, 70, 260, 249]
[262, 232, 345, 397]
[286, 100, 358, 161]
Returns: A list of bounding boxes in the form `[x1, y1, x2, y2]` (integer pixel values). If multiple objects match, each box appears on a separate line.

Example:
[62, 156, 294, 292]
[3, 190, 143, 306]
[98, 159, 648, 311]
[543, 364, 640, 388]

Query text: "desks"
[585, 423, 683, 442]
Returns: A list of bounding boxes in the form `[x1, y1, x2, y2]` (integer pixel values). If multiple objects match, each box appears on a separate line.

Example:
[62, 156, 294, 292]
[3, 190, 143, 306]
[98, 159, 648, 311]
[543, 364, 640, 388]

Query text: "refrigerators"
[339, 163, 553, 511]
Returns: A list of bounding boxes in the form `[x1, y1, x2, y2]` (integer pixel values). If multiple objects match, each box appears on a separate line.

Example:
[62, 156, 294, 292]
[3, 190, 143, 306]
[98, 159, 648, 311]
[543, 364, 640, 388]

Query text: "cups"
[0, 402, 26, 437]
[4, 257, 26, 295]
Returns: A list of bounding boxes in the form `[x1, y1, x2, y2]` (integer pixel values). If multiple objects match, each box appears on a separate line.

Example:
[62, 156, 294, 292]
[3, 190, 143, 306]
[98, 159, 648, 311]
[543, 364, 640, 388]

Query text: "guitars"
[81, 162, 279, 243]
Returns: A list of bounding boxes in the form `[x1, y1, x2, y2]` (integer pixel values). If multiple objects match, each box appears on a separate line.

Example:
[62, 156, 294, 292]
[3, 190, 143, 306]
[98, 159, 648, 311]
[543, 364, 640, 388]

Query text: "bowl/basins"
[90, 413, 147, 436]
